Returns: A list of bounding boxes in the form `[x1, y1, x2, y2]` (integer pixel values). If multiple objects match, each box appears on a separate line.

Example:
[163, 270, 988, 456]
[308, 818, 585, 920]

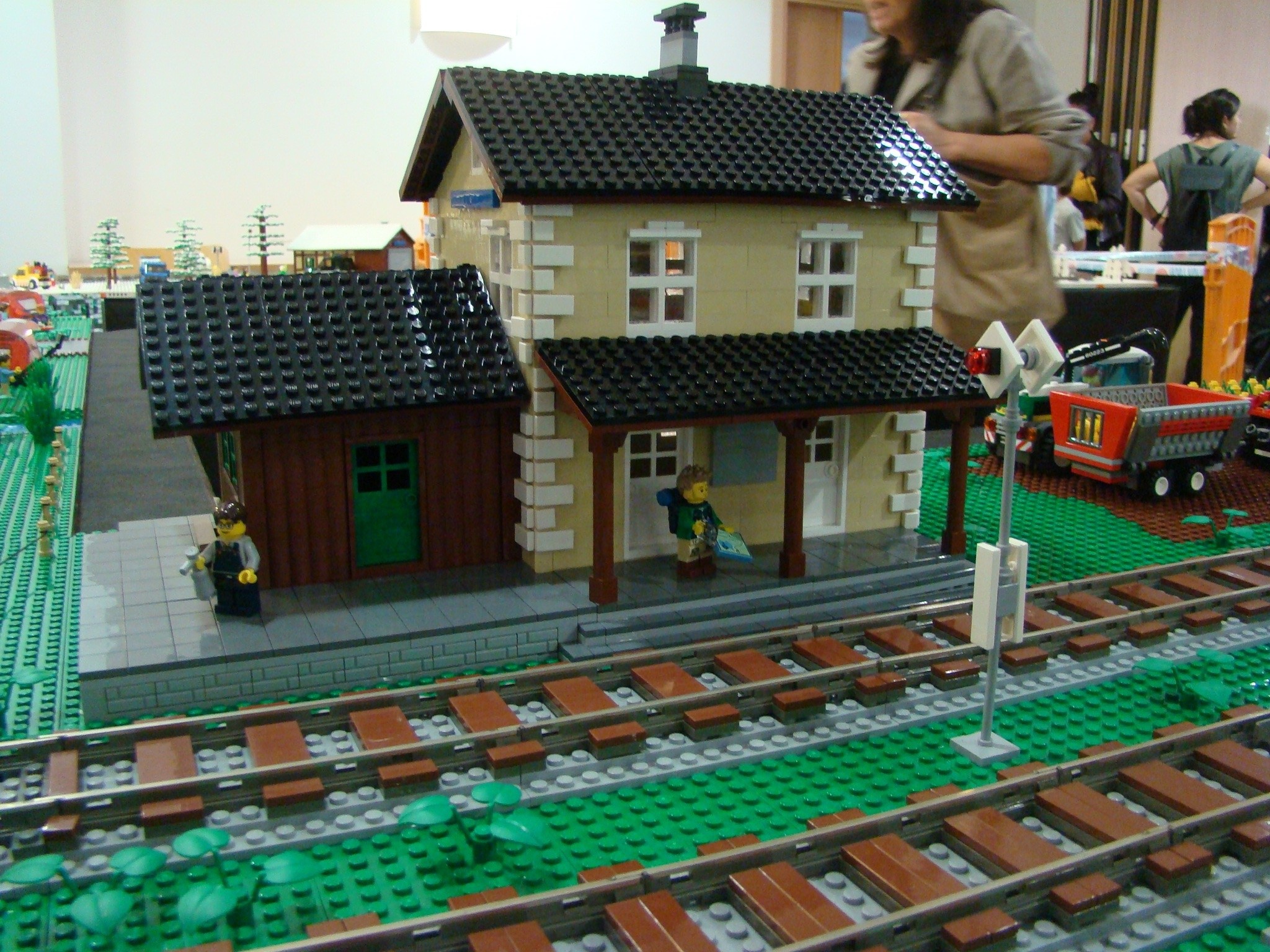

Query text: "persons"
[1120, 87, 1270, 386]
[848, 0, 1095, 353]
[1053, 82, 1124, 251]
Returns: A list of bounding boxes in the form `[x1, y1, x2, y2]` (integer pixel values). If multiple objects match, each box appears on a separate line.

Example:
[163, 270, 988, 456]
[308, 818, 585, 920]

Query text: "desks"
[1049, 286, 1195, 385]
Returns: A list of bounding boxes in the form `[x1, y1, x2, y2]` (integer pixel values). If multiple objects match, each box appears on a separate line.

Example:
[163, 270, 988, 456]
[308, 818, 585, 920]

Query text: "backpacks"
[1161, 144, 1242, 251]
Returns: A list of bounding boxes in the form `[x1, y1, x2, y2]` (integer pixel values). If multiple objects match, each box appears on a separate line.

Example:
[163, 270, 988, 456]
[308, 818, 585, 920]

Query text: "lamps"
[410, 0, 519, 41]
